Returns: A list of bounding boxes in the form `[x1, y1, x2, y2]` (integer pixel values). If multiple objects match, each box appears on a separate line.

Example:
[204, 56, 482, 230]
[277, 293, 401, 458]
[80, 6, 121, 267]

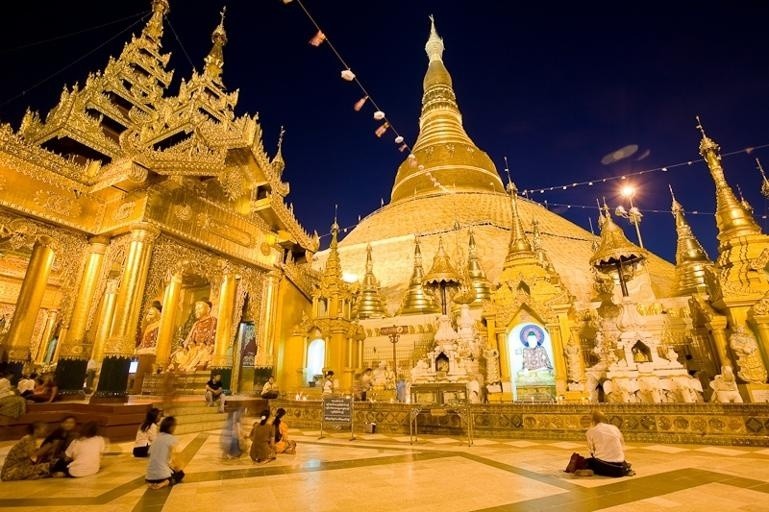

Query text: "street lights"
[620, 179, 645, 248]
[387, 323, 402, 392]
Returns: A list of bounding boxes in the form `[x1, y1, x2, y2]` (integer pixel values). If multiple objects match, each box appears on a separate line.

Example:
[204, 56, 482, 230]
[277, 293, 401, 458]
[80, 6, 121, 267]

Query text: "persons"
[205, 373, 225, 413]
[0, 368, 105, 479]
[261, 375, 278, 399]
[222, 408, 244, 458]
[144, 415, 184, 489]
[133, 407, 163, 457]
[522, 331, 553, 383]
[134, 300, 163, 353]
[372, 360, 395, 383]
[271, 408, 297, 453]
[584, 411, 631, 476]
[163, 295, 218, 371]
[249, 410, 278, 464]
[322, 370, 333, 393]
[353, 368, 374, 399]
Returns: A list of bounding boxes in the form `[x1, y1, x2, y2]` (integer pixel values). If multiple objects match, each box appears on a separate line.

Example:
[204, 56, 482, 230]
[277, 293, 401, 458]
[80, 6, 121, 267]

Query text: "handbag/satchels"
[565, 453, 585, 473]
[283, 439, 298, 455]
